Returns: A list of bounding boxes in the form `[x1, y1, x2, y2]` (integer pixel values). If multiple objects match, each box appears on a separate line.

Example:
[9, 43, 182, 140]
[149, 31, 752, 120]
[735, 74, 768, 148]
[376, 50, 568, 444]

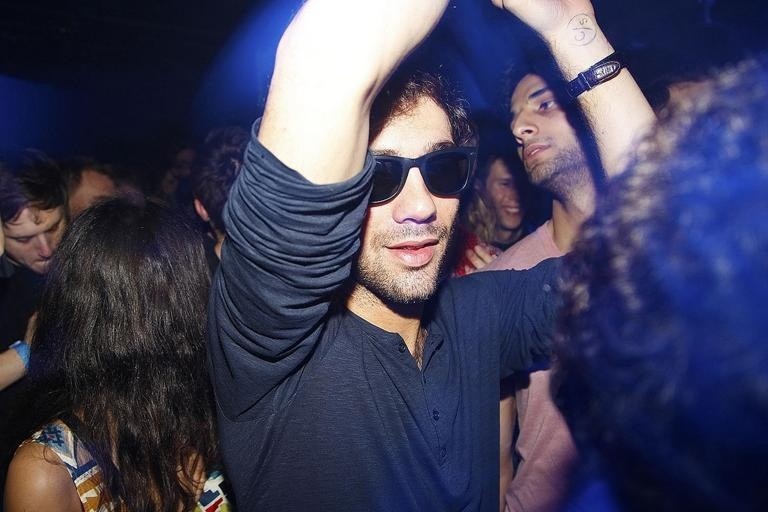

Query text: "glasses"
[368, 147, 481, 205]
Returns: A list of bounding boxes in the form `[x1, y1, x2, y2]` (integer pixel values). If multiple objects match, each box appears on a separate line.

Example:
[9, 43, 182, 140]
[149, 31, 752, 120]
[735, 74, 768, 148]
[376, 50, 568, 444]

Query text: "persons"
[1, 0, 768, 511]
